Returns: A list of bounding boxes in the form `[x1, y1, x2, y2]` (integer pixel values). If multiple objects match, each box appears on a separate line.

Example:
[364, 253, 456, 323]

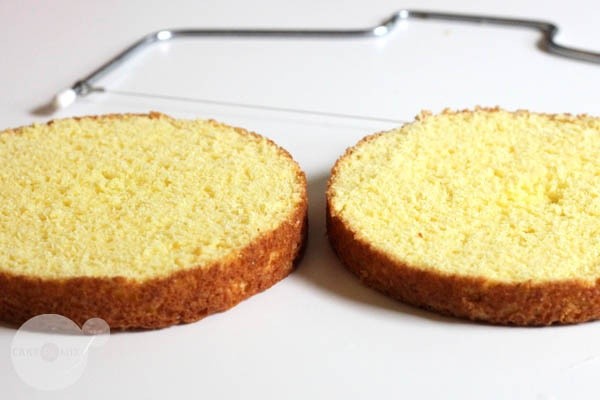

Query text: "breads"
[0, 112, 307, 334]
[326, 103, 597, 321]
